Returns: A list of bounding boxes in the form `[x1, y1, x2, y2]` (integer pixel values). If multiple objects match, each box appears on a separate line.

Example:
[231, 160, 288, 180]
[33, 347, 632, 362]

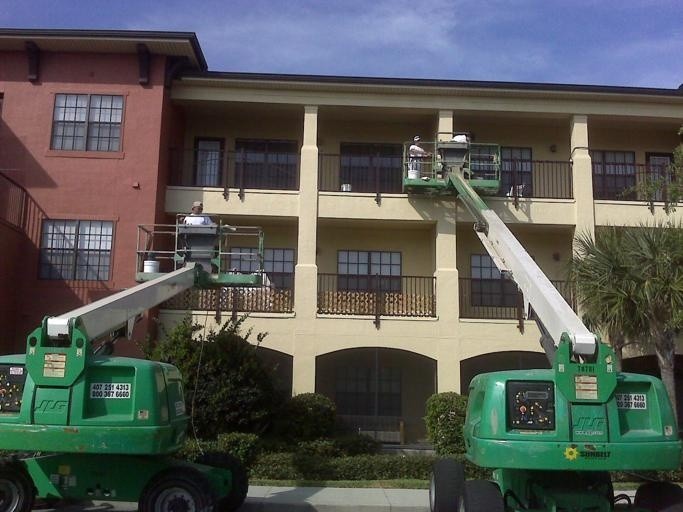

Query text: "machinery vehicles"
[0, 212, 265, 512]
[399, 135, 683, 512]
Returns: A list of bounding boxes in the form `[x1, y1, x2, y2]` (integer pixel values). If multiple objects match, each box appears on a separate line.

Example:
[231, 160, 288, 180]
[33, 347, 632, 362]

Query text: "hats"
[192, 201, 203, 208]
[414, 136, 420, 141]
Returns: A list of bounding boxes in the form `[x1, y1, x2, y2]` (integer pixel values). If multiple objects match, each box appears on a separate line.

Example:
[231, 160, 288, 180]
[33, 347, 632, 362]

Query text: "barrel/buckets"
[406, 169, 422, 180]
[142, 259, 160, 274]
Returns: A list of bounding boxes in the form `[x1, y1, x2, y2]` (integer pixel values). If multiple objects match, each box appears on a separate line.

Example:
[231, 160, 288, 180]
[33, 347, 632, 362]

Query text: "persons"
[408, 135, 430, 179]
[184, 200, 212, 226]
